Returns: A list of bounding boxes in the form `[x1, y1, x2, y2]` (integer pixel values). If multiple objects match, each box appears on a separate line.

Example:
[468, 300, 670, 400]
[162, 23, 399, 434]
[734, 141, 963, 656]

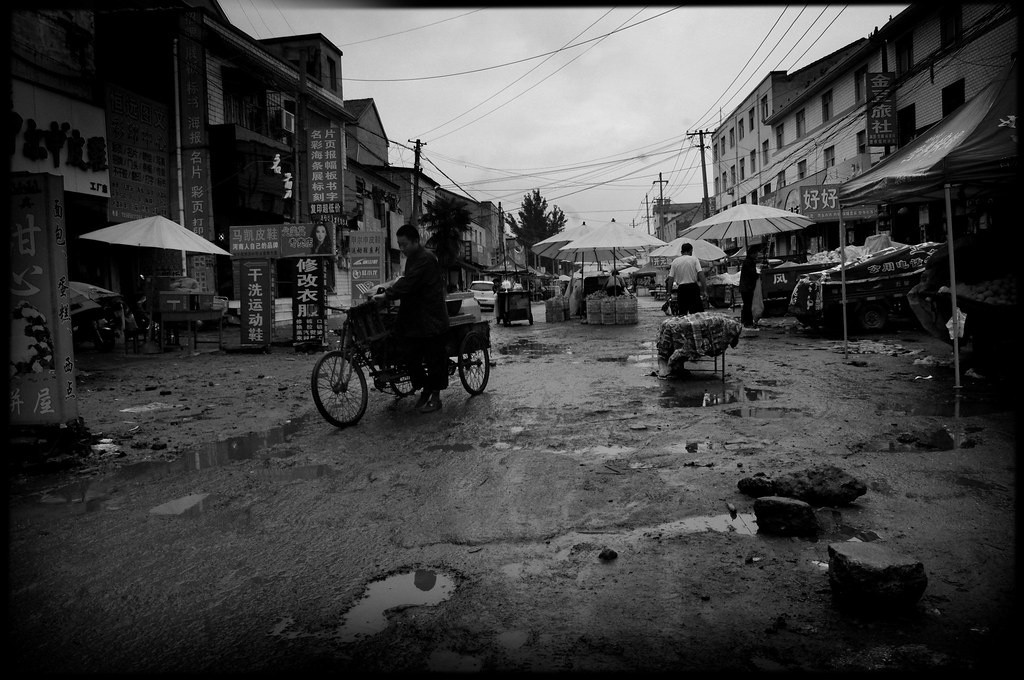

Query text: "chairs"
[122, 308, 148, 357]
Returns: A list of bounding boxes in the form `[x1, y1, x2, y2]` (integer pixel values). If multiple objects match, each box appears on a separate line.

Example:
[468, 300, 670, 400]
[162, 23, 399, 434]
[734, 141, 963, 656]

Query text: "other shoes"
[417, 390, 432, 407]
[422, 401, 443, 412]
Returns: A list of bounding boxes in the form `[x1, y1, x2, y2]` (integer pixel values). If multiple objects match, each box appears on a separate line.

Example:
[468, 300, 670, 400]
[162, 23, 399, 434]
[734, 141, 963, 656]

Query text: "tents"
[484, 255, 549, 290]
[838, 58, 1024, 389]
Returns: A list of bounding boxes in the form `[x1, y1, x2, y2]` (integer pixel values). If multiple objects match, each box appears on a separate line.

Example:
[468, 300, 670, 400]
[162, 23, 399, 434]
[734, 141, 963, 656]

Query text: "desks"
[662, 315, 728, 383]
[160, 310, 223, 351]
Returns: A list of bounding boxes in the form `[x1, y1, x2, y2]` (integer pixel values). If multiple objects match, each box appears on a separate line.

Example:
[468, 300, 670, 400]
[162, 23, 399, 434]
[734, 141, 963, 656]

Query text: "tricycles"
[307, 287, 494, 430]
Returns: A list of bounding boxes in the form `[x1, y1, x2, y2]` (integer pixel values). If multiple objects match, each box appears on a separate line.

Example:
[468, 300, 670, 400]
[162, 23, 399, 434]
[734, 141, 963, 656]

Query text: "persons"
[739, 247, 761, 331]
[602, 270, 625, 296]
[372, 225, 448, 411]
[668, 243, 707, 315]
[310, 223, 332, 254]
[492, 276, 523, 324]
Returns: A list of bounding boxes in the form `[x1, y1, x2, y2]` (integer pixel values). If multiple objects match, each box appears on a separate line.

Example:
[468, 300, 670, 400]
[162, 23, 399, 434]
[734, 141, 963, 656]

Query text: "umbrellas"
[648, 237, 727, 260]
[681, 204, 816, 254]
[78, 215, 233, 256]
[531, 218, 669, 295]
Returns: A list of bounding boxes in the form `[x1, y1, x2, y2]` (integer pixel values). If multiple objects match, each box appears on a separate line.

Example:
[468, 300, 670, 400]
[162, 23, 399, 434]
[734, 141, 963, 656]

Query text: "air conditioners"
[726, 187, 734, 195]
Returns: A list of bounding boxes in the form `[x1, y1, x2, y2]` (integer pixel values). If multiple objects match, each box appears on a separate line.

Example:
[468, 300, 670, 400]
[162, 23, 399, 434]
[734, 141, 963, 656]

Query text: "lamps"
[275, 108, 295, 133]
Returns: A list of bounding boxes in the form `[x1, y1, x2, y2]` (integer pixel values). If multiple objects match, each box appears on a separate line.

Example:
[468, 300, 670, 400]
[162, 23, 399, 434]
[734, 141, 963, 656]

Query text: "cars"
[466, 280, 497, 309]
[789, 241, 949, 335]
[759, 231, 897, 317]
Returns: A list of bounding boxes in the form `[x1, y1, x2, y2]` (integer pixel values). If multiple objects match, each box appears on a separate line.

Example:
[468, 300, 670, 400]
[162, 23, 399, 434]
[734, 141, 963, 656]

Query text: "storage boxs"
[616, 300, 637, 313]
[586, 312, 601, 324]
[546, 297, 570, 309]
[159, 293, 215, 310]
[616, 311, 639, 324]
[600, 300, 616, 313]
[546, 308, 570, 322]
[601, 313, 615, 324]
[586, 297, 601, 313]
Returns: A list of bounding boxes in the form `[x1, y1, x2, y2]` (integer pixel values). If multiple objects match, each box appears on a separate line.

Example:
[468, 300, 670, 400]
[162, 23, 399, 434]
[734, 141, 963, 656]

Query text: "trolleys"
[667, 288, 709, 315]
